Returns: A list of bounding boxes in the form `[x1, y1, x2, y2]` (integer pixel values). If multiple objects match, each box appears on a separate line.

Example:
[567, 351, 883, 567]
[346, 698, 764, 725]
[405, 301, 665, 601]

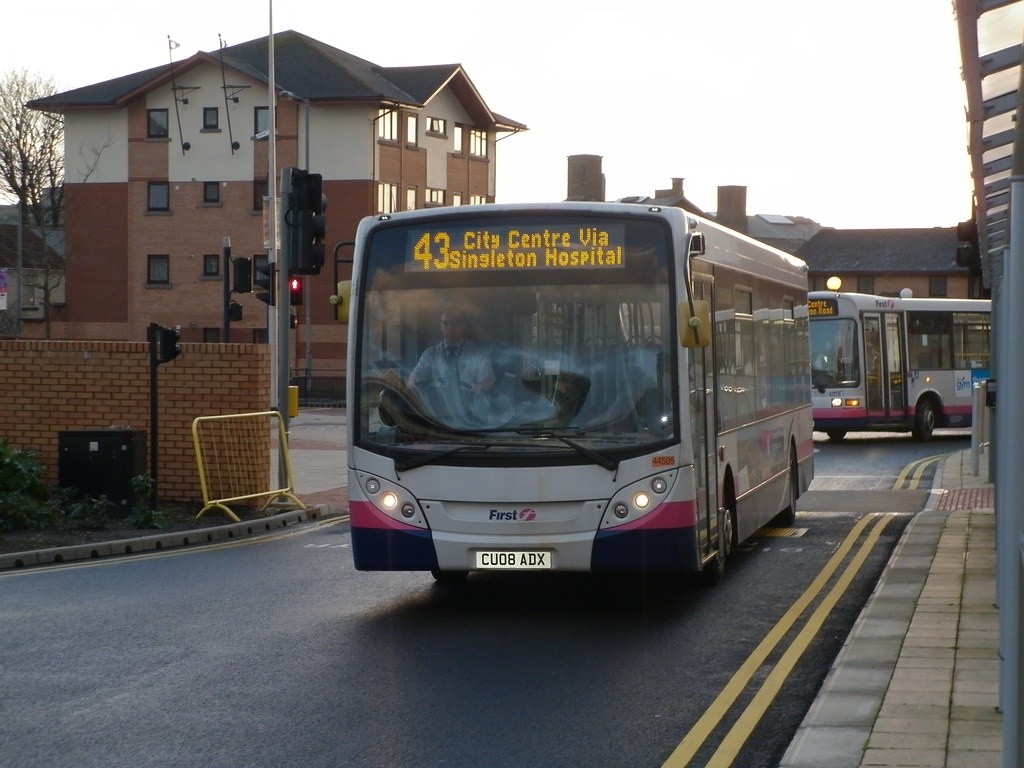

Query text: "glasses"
[440, 319, 464, 326]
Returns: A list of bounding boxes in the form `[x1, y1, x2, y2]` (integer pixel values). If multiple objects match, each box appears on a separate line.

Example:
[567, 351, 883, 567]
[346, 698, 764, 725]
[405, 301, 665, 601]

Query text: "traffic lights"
[160, 328, 184, 359]
[289, 277, 304, 305]
[293, 169, 329, 275]
[255, 260, 274, 305]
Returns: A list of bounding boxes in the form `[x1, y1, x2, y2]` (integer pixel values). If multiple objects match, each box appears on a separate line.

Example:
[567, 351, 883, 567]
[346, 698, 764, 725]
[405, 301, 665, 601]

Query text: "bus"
[328, 200, 818, 596]
[808, 289, 993, 441]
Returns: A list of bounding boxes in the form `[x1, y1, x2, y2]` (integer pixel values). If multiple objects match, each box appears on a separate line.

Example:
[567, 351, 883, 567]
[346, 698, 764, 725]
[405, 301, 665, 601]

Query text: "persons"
[405, 308, 495, 399]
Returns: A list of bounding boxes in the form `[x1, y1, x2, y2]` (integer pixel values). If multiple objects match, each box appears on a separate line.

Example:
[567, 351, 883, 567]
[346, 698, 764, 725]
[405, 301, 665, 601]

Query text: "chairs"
[584, 336, 664, 385]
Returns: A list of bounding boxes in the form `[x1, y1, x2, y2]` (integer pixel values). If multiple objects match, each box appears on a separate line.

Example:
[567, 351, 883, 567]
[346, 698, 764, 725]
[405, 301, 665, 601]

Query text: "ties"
[449, 348, 456, 361]
